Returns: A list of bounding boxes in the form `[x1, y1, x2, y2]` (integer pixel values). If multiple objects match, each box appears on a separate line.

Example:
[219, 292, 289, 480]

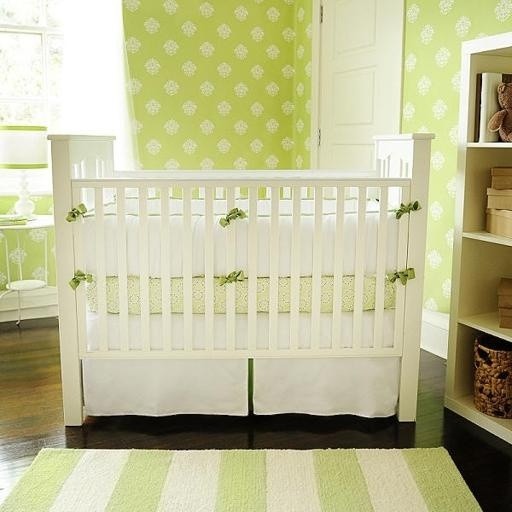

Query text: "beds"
[47, 133, 435, 427]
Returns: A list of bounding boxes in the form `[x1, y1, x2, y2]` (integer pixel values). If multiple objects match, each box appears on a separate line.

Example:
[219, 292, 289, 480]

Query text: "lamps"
[0, 125, 47, 218]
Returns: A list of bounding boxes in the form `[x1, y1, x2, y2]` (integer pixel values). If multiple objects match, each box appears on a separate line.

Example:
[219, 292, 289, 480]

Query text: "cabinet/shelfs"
[443, 32, 511, 446]
[0, 214, 55, 325]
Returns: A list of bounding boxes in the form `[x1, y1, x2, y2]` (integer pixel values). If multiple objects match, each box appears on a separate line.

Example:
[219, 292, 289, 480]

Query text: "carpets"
[0, 448, 484, 512]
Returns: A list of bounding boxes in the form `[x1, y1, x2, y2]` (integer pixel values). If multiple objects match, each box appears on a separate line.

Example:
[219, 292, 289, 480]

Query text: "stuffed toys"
[488, 82, 512, 143]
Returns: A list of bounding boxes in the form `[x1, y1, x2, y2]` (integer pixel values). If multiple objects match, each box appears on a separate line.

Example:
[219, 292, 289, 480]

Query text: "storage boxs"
[485, 167, 512, 242]
[498, 308, 512, 328]
[496, 277, 512, 308]
